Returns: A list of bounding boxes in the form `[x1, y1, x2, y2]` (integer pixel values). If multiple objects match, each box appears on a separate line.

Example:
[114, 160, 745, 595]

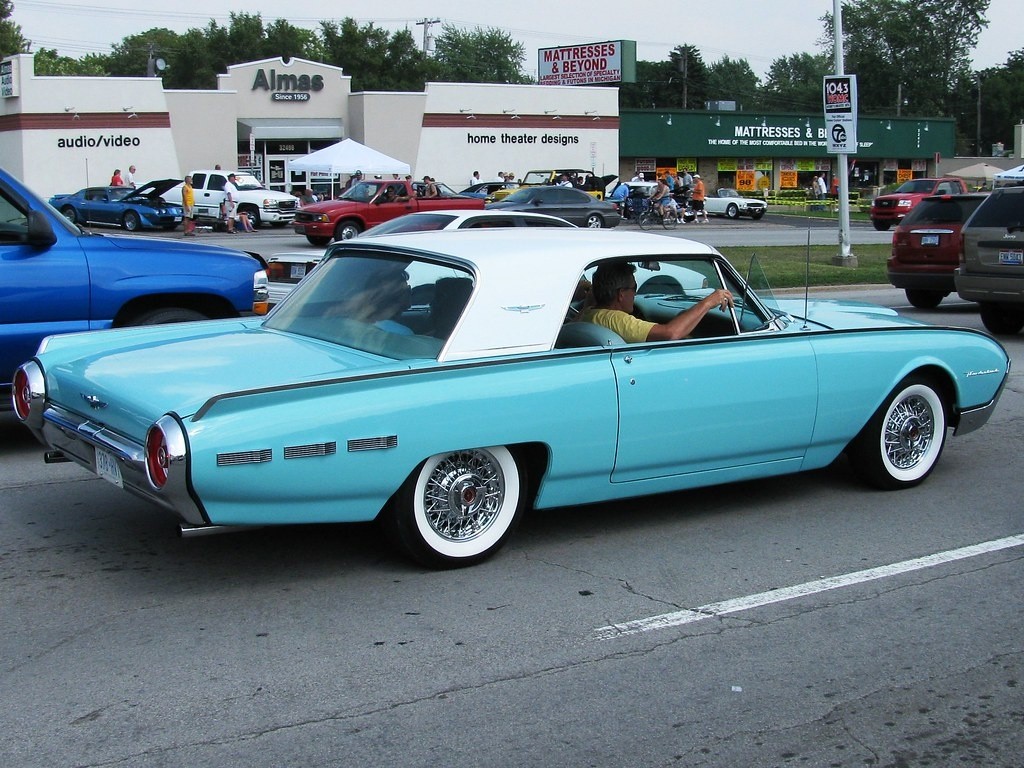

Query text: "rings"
[724, 297, 727, 300]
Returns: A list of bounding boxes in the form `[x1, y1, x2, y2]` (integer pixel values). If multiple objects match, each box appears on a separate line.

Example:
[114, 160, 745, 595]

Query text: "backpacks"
[429, 183, 441, 197]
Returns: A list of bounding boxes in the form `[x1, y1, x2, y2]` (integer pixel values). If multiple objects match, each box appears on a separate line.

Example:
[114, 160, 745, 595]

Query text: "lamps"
[755, 116, 767, 127]
[710, 115, 721, 127]
[918, 121, 929, 132]
[585, 109, 601, 121]
[544, 109, 561, 120]
[460, 108, 476, 120]
[123, 107, 138, 120]
[503, 108, 521, 120]
[65, 105, 80, 122]
[660, 114, 672, 126]
[798, 117, 811, 128]
[880, 120, 892, 130]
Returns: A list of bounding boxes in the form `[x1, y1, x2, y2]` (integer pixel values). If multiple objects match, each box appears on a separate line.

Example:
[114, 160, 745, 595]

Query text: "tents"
[992, 164, 1024, 190]
[944, 162, 1005, 193]
[288, 138, 411, 200]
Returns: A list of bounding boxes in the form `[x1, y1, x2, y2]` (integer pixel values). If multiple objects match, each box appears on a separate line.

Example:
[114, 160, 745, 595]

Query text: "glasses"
[623, 283, 637, 290]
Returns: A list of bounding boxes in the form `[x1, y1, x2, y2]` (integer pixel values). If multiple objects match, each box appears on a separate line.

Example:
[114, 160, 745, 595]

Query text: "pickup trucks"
[869, 177, 968, 232]
[128, 170, 301, 231]
[295, 180, 485, 247]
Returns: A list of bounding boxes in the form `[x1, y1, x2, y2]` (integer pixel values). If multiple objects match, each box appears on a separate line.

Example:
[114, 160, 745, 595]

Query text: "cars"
[457, 168, 621, 212]
[885, 192, 992, 308]
[11, 225, 1011, 572]
[267, 209, 709, 325]
[409, 180, 467, 199]
[954, 186, 1024, 335]
[622, 181, 708, 225]
[48, 178, 185, 232]
[486, 185, 620, 229]
[0, 168, 271, 411]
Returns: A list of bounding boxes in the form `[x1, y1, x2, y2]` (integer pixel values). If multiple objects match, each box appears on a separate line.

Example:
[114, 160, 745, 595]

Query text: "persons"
[555, 173, 584, 188]
[215, 164, 221, 170]
[472, 171, 483, 186]
[495, 171, 515, 183]
[125, 165, 136, 189]
[290, 188, 331, 205]
[570, 261, 734, 344]
[182, 175, 195, 236]
[112, 169, 123, 186]
[224, 173, 259, 234]
[978, 180, 1024, 192]
[808, 172, 839, 212]
[610, 168, 710, 224]
[363, 267, 474, 340]
[334, 170, 437, 204]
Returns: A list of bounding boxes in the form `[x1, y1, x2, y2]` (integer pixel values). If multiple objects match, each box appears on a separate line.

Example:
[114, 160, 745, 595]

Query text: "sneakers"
[690, 219, 699, 223]
[701, 219, 710, 224]
[676, 218, 686, 224]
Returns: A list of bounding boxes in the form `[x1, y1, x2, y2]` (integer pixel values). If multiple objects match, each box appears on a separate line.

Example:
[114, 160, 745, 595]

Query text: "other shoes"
[245, 230, 250, 233]
[251, 230, 258, 232]
[621, 216, 628, 219]
[228, 229, 239, 234]
[185, 232, 195, 236]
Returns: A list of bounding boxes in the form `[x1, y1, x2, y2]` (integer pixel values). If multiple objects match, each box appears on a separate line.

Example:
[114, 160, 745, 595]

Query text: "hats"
[422, 176, 430, 180]
[693, 174, 701, 179]
[639, 173, 644, 178]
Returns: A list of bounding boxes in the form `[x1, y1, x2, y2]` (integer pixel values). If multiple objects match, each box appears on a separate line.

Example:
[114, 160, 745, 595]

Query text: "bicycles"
[638, 200, 678, 232]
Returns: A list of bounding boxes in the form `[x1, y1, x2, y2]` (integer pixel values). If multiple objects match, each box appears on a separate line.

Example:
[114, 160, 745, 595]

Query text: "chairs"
[430, 278, 473, 340]
[937, 203, 963, 223]
[554, 321, 626, 349]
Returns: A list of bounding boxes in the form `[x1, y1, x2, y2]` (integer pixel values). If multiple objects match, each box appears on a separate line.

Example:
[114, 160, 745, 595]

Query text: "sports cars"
[687, 188, 768, 220]
